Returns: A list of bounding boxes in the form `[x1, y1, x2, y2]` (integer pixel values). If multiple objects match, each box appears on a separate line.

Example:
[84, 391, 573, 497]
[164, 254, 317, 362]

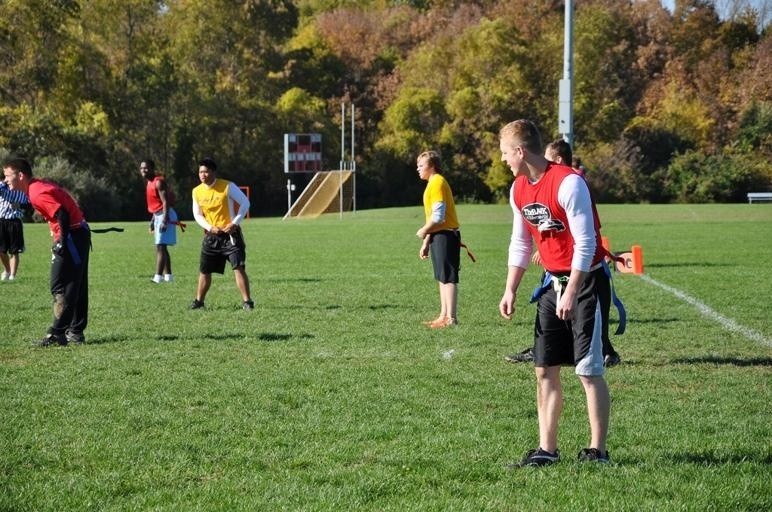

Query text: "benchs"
[747, 192, 772, 204]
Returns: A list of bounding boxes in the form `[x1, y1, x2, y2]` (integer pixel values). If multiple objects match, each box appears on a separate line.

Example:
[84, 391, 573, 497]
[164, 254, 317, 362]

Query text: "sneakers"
[506, 349, 534, 362]
[421, 317, 457, 328]
[602, 352, 620, 367]
[188, 300, 204, 310]
[243, 301, 253, 310]
[509, 448, 560, 468]
[577, 448, 610, 464]
[1, 271, 9, 281]
[37, 331, 84, 347]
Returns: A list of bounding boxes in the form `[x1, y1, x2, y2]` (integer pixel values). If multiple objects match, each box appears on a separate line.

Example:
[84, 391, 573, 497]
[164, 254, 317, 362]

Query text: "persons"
[416, 150, 475, 328]
[498, 119, 610, 471]
[0, 182, 30, 281]
[504, 139, 621, 368]
[188, 159, 253, 312]
[139, 159, 186, 283]
[3, 159, 123, 347]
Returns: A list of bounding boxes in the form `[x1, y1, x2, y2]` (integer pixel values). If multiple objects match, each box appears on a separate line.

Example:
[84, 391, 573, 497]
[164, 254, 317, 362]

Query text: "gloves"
[51, 241, 65, 255]
